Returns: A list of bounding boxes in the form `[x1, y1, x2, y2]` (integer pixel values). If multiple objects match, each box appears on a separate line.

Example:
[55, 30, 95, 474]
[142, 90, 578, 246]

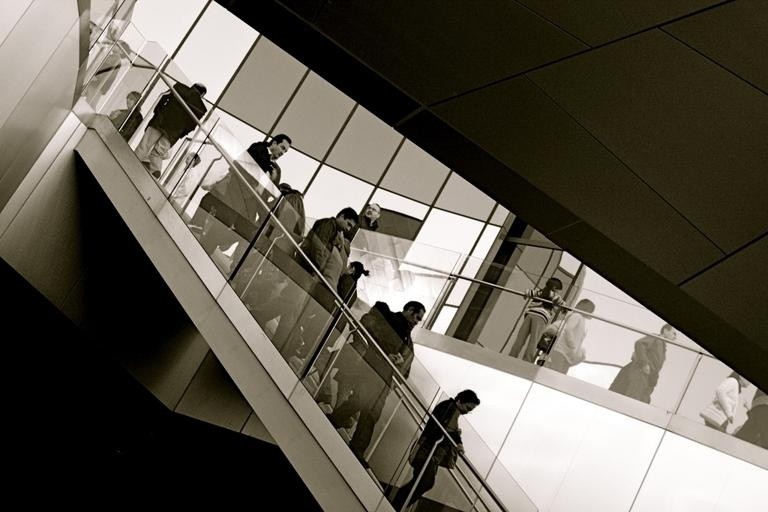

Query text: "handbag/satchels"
[699, 402, 728, 429]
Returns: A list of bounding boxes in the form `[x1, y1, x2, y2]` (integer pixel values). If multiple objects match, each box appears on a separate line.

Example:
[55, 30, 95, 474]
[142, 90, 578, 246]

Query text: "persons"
[607, 350, 639, 396]
[348, 201, 382, 266]
[313, 299, 392, 430]
[507, 276, 570, 360]
[108, 91, 146, 142]
[81, 38, 132, 112]
[541, 296, 597, 375]
[320, 300, 428, 473]
[131, 82, 209, 183]
[164, 151, 201, 200]
[622, 323, 679, 406]
[185, 160, 372, 366]
[701, 367, 754, 435]
[380, 387, 483, 512]
[184, 132, 293, 255]
[732, 386, 768, 451]
